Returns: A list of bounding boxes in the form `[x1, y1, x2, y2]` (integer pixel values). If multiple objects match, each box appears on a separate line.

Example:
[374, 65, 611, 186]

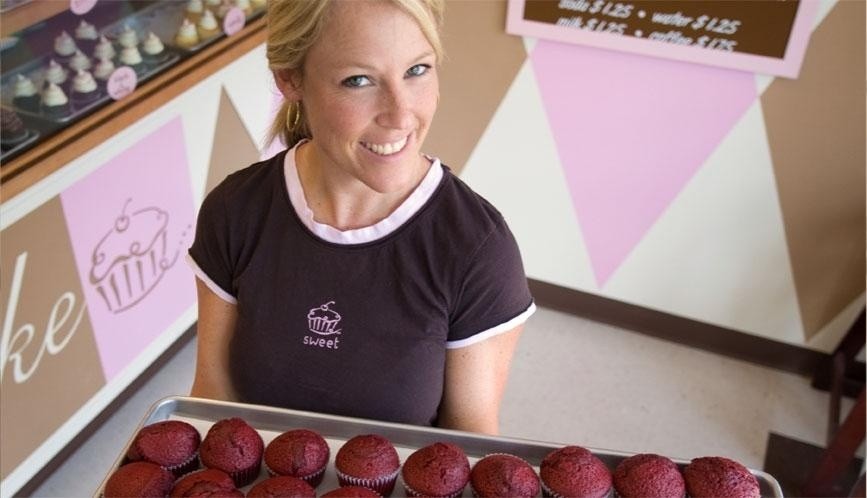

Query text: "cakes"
[0, 0, 270, 144]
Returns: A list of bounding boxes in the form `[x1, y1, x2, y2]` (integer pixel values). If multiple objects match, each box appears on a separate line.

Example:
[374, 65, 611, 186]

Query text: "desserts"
[105, 416, 762, 497]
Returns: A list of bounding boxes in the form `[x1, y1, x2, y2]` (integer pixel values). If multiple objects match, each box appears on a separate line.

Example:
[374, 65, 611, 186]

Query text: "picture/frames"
[504, 0, 818, 80]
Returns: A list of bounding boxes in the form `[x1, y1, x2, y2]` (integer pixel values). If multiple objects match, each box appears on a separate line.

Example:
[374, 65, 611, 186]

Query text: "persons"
[185, 0, 538, 439]
